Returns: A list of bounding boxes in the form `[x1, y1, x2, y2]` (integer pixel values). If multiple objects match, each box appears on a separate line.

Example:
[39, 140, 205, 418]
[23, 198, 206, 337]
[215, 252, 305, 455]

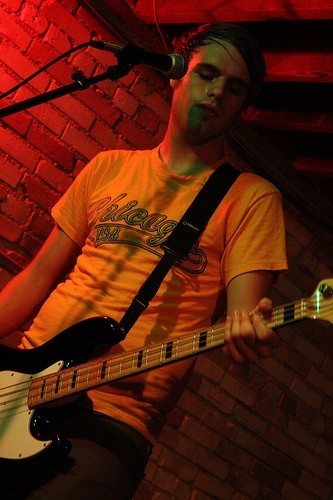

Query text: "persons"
[0, 22, 291, 500]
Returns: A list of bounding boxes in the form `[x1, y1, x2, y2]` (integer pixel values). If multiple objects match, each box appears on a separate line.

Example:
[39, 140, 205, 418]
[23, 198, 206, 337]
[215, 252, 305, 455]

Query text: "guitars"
[0, 277, 332, 475]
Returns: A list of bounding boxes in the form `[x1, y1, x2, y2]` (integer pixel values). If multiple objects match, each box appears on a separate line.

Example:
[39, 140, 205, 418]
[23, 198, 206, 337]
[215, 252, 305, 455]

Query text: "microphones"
[90, 40, 189, 80]
